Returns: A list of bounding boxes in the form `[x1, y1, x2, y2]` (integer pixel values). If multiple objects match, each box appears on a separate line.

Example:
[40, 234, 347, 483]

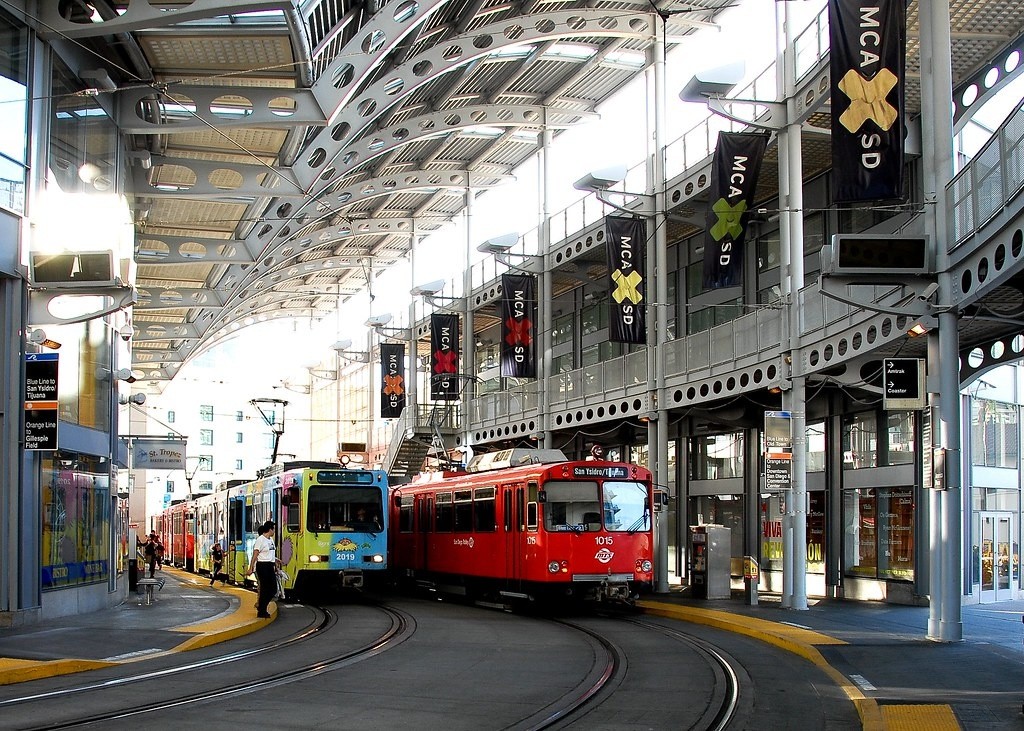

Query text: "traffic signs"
[883, 358, 920, 400]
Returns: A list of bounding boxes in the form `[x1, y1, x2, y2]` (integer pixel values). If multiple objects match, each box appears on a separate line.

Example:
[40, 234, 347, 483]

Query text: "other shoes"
[221, 581, 226, 587]
[209, 584, 212, 587]
[258, 611, 271, 618]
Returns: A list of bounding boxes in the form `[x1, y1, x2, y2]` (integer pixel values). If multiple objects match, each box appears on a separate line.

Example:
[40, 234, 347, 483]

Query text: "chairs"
[583, 512, 601, 530]
[133, 566, 165, 606]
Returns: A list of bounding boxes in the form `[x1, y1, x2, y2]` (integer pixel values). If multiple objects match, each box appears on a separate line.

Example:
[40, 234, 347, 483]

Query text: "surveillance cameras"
[917, 282, 940, 302]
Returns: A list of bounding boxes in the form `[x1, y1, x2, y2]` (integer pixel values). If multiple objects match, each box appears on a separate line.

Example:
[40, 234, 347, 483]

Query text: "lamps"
[32, 329, 62, 350]
[530, 432, 544, 441]
[455, 445, 465, 454]
[116, 368, 136, 384]
[767, 381, 792, 394]
[907, 315, 938, 339]
[130, 392, 146, 405]
[637, 412, 659, 423]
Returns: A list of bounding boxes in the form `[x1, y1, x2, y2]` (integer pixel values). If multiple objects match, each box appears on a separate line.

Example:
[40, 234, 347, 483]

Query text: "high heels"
[254, 603, 259, 609]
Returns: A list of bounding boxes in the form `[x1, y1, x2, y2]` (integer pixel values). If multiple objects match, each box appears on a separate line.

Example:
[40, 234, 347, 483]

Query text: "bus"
[388, 447, 672, 614]
[156, 460, 403, 602]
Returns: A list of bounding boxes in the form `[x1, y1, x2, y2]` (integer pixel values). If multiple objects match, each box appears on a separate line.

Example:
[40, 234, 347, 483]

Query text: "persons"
[209, 543, 229, 586]
[247, 521, 282, 618]
[136, 530, 165, 579]
